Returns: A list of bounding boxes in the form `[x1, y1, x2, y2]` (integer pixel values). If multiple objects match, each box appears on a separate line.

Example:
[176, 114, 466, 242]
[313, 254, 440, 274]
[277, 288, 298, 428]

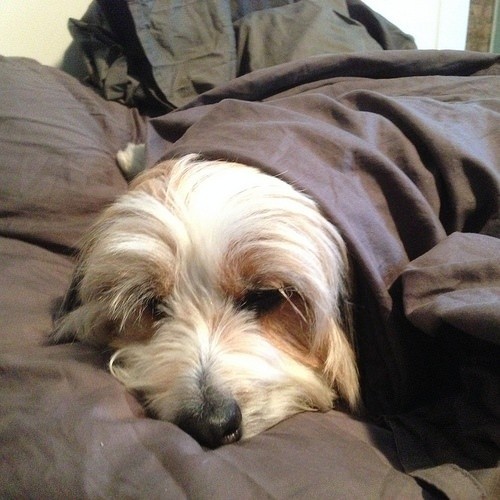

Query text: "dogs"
[46, 151, 365, 450]
